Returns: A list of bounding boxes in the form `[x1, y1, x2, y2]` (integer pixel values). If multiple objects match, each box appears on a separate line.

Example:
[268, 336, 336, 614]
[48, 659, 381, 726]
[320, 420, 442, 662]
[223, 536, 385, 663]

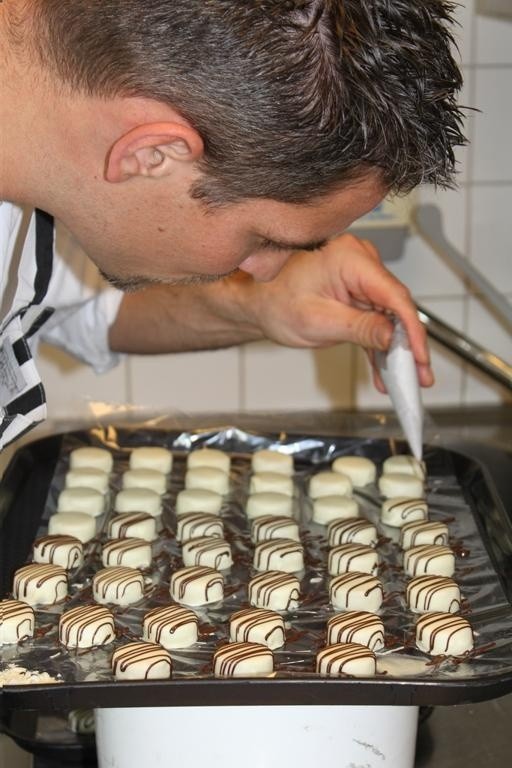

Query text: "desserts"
[1, 446, 475, 680]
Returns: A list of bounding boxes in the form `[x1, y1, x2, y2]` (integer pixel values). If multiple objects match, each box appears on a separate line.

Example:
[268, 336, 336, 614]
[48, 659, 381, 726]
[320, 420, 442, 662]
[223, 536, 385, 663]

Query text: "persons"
[0, 1, 484, 458]
